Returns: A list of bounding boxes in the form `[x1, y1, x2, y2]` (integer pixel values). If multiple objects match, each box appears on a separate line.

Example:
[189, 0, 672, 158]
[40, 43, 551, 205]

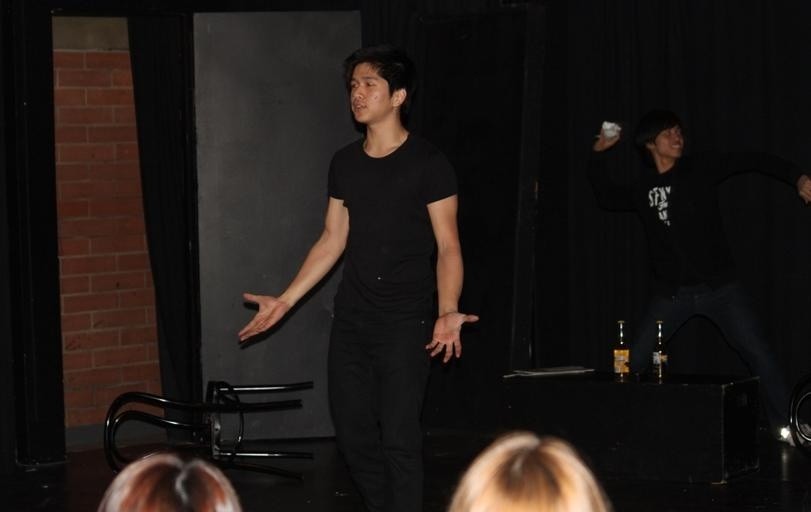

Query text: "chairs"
[101, 381, 324, 488]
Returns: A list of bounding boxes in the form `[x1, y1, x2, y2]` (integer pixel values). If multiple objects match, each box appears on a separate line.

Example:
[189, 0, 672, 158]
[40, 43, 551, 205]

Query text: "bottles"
[613, 321, 631, 384]
[649, 320, 668, 386]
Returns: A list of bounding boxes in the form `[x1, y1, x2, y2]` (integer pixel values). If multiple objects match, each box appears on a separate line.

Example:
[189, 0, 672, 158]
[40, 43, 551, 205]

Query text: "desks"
[494, 373, 759, 489]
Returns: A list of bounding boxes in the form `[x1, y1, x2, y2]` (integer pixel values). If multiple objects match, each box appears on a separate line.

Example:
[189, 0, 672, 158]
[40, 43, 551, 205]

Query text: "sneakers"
[776, 416, 811, 448]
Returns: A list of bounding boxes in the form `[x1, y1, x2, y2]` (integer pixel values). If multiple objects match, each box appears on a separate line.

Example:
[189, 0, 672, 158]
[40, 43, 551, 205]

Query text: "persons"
[235, 42, 484, 510]
[447, 429, 616, 511]
[97, 450, 245, 512]
[590, 110, 810, 434]
